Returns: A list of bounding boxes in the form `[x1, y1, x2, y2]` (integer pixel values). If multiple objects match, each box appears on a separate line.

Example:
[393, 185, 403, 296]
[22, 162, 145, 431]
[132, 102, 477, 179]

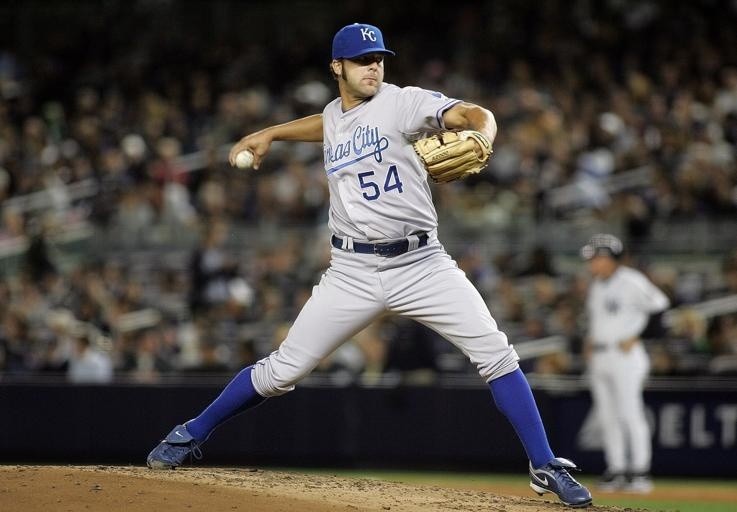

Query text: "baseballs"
[236, 150, 254, 170]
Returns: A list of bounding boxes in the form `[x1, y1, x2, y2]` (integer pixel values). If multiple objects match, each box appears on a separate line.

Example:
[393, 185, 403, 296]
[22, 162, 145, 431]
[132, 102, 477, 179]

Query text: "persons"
[581, 232, 671, 494]
[148, 21, 592, 507]
[1, 1, 737, 388]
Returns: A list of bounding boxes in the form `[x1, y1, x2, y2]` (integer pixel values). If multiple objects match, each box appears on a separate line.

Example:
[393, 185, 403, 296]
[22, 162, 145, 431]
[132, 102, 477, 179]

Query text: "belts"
[332, 232, 427, 258]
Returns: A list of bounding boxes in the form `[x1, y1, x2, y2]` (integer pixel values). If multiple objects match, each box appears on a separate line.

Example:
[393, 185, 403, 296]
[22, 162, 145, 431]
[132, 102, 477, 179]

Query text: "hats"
[331, 23, 396, 61]
[580, 232, 622, 259]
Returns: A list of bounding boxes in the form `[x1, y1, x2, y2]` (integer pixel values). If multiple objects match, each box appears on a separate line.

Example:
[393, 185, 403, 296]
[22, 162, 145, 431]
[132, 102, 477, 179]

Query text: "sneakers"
[146, 418, 205, 470]
[528, 456, 592, 508]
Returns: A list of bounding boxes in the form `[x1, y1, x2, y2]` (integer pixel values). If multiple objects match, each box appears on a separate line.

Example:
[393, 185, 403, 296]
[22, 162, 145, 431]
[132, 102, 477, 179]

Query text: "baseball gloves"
[411, 127, 493, 185]
